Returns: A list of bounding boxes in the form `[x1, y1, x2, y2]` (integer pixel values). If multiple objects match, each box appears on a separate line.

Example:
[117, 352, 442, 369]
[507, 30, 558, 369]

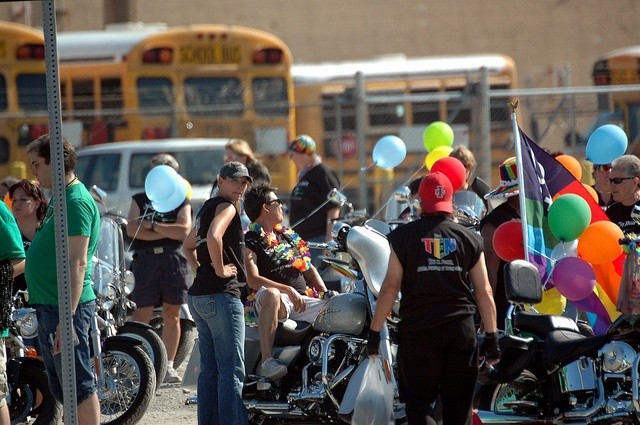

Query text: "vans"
[71, 137, 239, 220]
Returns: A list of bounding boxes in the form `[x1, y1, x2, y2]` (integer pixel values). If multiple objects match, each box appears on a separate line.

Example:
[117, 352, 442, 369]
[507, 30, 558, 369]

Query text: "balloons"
[425, 145, 455, 172]
[555, 155, 583, 182]
[553, 257, 596, 301]
[372, 135, 407, 169]
[582, 184, 598, 204]
[585, 123, 628, 164]
[548, 193, 592, 242]
[577, 221, 625, 265]
[424, 121, 455, 152]
[430, 157, 467, 192]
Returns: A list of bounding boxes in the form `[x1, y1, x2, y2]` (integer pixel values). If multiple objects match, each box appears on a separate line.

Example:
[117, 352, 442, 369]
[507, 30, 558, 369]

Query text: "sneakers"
[261, 358, 288, 380]
[163, 367, 181, 382]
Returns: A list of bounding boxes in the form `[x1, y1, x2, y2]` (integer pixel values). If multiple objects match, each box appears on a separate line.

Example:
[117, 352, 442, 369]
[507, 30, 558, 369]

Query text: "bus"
[0, 19, 49, 185]
[55, 22, 296, 199]
[294, 55, 519, 223]
[592, 46, 639, 155]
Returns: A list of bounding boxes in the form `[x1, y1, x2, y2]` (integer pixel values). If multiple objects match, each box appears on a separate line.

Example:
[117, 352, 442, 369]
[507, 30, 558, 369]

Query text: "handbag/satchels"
[615, 239, 640, 315]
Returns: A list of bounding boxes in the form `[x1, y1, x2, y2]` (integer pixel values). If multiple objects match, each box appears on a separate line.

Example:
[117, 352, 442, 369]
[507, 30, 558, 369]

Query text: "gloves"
[366, 329, 381, 356]
[479, 332, 502, 360]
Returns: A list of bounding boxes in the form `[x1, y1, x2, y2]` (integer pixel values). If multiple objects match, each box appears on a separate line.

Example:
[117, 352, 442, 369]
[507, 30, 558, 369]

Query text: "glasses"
[602, 165, 612, 169]
[609, 178, 635, 184]
[267, 199, 281, 204]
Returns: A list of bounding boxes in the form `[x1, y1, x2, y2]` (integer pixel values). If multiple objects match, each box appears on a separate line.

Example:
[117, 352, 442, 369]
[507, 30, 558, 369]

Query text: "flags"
[518, 125, 629, 337]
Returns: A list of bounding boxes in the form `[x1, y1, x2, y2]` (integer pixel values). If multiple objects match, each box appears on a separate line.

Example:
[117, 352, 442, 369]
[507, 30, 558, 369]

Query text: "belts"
[140, 245, 177, 255]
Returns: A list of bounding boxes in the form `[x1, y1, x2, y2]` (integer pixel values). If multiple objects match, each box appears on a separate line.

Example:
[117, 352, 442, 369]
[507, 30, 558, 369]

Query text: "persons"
[24, 135, 103, 424]
[589, 161, 615, 209]
[397, 179, 420, 225]
[361, 172, 501, 425]
[8, 179, 49, 250]
[126, 154, 191, 384]
[0, 197, 28, 425]
[241, 184, 341, 380]
[446, 144, 476, 190]
[209, 140, 272, 214]
[474, 157, 523, 330]
[604, 153, 640, 248]
[181, 162, 253, 425]
[285, 135, 343, 269]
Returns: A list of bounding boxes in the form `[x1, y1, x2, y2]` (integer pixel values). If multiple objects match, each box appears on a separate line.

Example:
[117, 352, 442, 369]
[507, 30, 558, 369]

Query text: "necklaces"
[38, 176, 79, 231]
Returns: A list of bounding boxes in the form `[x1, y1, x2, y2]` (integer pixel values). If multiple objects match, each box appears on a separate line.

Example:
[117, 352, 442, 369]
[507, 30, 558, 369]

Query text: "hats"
[484, 157, 519, 200]
[419, 171, 453, 214]
[220, 162, 253, 183]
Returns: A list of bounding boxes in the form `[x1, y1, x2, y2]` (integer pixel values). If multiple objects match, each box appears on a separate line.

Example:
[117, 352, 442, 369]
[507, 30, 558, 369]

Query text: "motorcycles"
[91, 182, 168, 411]
[244, 217, 442, 425]
[90, 217, 157, 425]
[305, 184, 414, 294]
[107, 207, 195, 373]
[472, 257, 640, 425]
[5, 287, 64, 425]
[410, 176, 495, 226]
